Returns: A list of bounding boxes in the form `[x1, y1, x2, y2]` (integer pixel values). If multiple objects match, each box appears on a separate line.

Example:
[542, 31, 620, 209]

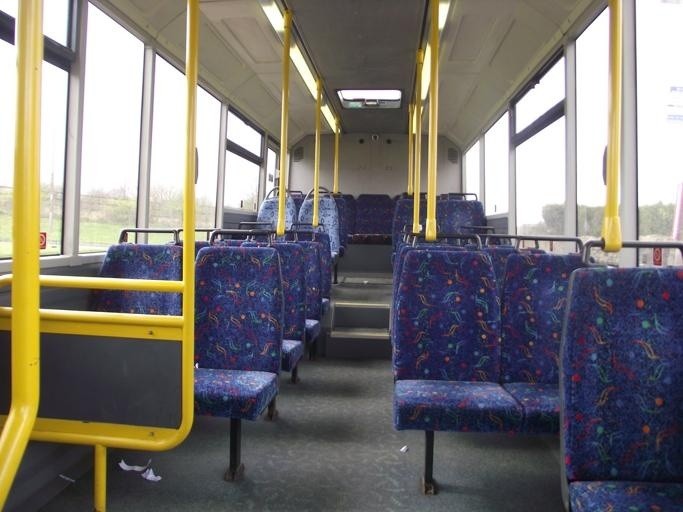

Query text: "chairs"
[77, 186, 352, 482]
[354, 193, 682, 512]
